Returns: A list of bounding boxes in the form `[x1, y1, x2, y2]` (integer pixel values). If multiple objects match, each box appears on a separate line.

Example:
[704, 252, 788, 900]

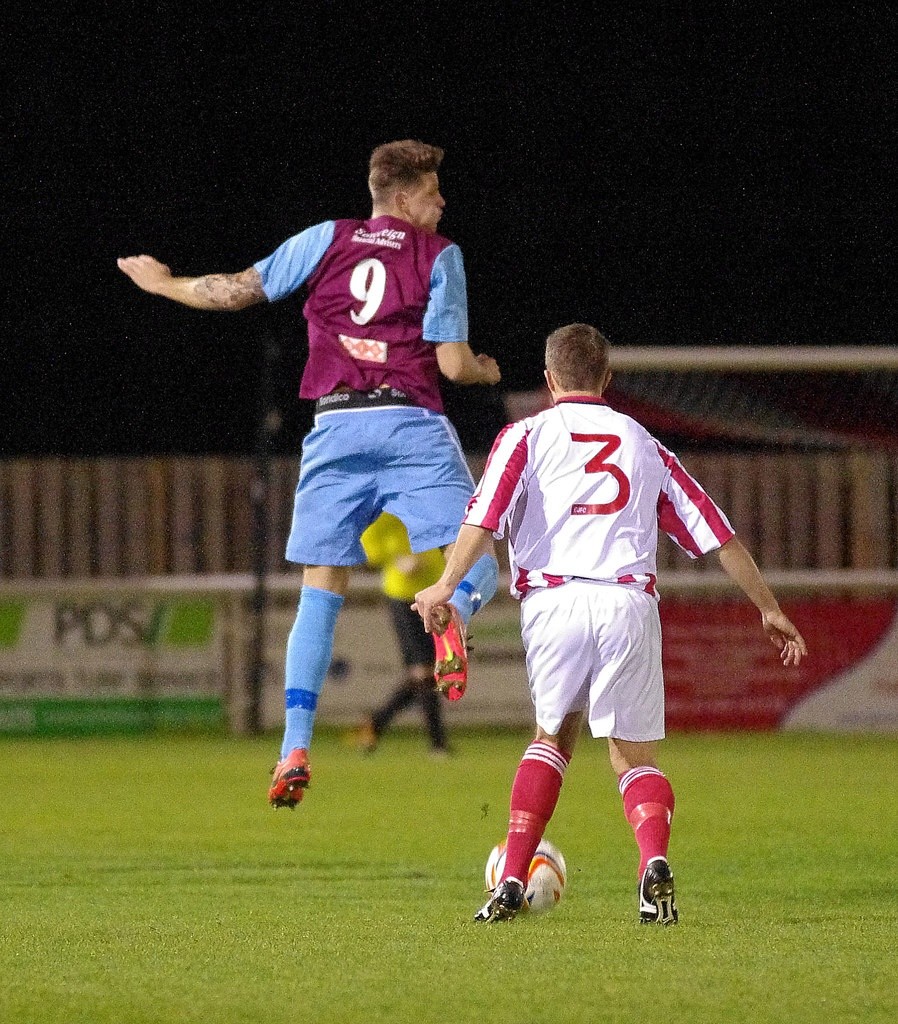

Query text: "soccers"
[484, 836, 568, 910]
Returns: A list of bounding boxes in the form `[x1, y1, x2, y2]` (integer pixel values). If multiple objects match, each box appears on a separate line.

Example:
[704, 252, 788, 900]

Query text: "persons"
[116, 138, 500, 811]
[410, 323, 808, 925]
[359, 512, 453, 762]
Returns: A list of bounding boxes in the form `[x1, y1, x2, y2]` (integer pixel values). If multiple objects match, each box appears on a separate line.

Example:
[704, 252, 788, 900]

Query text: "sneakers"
[430, 604, 468, 702]
[637, 856, 680, 926]
[268, 748, 311, 811]
[476, 877, 526, 923]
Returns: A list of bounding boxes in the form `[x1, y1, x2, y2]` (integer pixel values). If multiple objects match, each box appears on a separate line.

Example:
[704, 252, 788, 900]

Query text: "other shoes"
[360, 719, 376, 754]
